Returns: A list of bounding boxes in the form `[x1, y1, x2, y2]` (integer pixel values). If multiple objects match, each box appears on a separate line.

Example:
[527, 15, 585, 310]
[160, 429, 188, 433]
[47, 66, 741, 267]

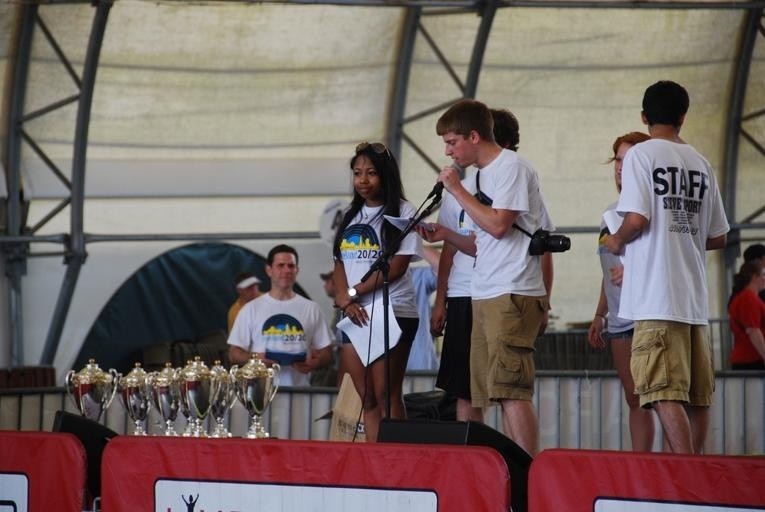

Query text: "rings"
[358, 306, 365, 311]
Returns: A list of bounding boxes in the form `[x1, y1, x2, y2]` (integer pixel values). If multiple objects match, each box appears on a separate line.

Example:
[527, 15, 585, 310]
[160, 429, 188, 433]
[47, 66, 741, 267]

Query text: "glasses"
[356, 143, 391, 158]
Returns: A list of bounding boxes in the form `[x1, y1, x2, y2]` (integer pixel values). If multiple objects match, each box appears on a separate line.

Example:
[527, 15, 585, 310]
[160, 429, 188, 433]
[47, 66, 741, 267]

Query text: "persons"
[429, 109, 554, 424]
[726, 245, 765, 309]
[414, 100, 550, 459]
[331, 142, 425, 442]
[602, 78, 731, 454]
[726, 258, 765, 370]
[226, 244, 441, 386]
[588, 130, 693, 453]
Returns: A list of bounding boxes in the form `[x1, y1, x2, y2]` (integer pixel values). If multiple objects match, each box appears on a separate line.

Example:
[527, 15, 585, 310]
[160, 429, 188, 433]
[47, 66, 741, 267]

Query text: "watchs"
[346, 287, 359, 301]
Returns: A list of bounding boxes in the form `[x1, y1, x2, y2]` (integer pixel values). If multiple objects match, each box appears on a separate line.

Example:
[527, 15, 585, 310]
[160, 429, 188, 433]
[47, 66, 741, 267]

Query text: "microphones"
[428, 161, 465, 199]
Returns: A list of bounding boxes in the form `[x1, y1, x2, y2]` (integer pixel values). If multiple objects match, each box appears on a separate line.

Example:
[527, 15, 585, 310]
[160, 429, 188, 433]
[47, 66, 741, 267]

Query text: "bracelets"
[595, 313, 608, 321]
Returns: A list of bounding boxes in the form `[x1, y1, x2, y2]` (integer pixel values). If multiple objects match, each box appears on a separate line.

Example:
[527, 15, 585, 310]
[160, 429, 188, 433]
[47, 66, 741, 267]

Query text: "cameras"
[528, 228, 570, 256]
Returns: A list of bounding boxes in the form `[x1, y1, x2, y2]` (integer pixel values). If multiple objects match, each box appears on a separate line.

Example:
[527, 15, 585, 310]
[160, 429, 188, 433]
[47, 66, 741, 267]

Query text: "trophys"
[64, 353, 281, 439]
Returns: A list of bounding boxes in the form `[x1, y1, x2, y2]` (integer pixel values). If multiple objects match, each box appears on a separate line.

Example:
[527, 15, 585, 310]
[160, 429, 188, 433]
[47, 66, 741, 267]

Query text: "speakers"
[376, 418, 532, 511]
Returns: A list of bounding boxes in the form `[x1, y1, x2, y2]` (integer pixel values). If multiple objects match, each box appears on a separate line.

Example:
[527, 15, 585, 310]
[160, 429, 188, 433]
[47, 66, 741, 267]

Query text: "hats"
[321, 272, 333, 280]
[234, 274, 260, 289]
